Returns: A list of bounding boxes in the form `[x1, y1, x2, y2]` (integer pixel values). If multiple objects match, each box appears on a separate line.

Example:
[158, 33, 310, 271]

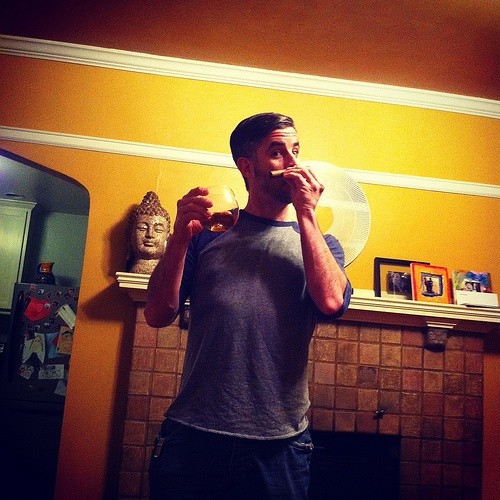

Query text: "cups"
[199, 185, 239, 232]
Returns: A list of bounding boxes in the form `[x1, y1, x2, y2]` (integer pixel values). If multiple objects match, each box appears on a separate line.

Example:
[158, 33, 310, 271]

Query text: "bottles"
[36, 262, 55, 285]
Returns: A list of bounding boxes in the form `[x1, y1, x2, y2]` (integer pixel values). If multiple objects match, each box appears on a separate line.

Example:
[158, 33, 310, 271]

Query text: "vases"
[451, 271, 491, 304]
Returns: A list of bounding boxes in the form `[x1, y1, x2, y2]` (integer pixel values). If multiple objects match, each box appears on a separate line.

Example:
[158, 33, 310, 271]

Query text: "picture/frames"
[410, 263, 451, 305]
[374, 257, 430, 300]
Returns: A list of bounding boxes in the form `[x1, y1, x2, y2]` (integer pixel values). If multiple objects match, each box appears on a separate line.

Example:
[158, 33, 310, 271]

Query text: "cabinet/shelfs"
[0, 198, 39, 314]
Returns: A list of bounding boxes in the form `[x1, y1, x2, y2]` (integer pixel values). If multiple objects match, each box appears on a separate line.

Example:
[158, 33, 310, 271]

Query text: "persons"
[144, 113, 353, 499]
[126, 191, 170, 274]
